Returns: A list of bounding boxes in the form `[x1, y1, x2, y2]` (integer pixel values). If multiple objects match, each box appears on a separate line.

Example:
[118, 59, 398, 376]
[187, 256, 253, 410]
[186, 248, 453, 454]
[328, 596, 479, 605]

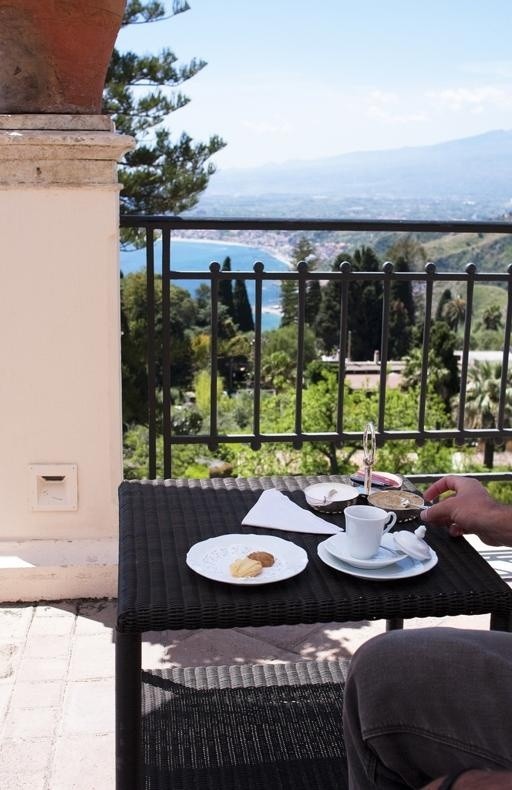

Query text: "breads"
[231, 558, 262, 577]
[247, 552, 274, 566]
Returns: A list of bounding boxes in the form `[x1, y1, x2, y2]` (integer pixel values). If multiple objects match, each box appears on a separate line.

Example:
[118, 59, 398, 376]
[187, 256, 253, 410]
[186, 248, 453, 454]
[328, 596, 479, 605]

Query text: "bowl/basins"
[303, 483, 359, 515]
[367, 490, 424, 523]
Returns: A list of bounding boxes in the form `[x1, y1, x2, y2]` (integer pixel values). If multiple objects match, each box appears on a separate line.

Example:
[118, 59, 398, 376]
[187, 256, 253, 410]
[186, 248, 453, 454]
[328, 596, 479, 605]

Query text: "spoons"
[393, 494, 432, 511]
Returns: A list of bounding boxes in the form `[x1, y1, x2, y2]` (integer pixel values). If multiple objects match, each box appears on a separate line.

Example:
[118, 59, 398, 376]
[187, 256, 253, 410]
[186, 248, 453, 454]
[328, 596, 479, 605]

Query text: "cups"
[344, 504, 398, 558]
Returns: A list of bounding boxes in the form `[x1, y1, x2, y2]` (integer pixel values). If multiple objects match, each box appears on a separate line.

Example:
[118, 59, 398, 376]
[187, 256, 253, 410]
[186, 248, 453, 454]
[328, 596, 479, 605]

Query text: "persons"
[343, 471, 511, 789]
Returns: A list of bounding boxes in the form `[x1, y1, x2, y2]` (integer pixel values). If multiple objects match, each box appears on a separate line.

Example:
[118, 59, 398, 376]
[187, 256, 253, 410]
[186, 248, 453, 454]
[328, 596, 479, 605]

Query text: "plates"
[316, 531, 440, 580]
[185, 533, 309, 587]
